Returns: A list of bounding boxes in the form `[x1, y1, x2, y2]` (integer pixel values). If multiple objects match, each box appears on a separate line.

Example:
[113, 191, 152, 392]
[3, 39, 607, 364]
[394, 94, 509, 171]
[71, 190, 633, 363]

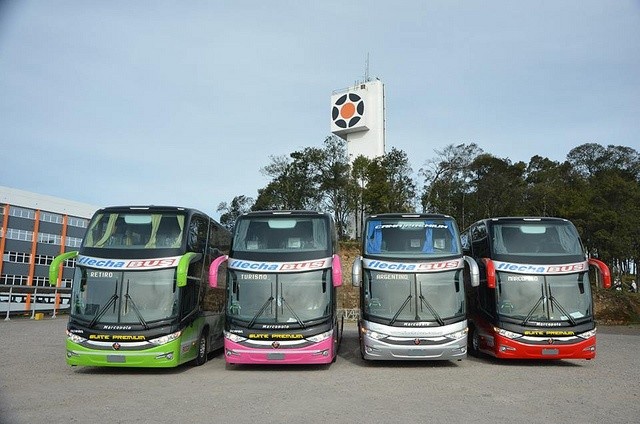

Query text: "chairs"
[243, 240, 263, 250]
[403, 236, 424, 250]
[371, 238, 387, 250]
[432, 235, 451, 251]
[281, 237, 305, 250]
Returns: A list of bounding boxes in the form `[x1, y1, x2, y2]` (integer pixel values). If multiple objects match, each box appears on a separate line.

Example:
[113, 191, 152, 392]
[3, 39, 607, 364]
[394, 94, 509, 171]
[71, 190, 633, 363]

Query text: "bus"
[350, 211, 480, 361]
[208, 203, 344, 364]
[49, 204, 231, 370]
[457, 215, 611, 360]
